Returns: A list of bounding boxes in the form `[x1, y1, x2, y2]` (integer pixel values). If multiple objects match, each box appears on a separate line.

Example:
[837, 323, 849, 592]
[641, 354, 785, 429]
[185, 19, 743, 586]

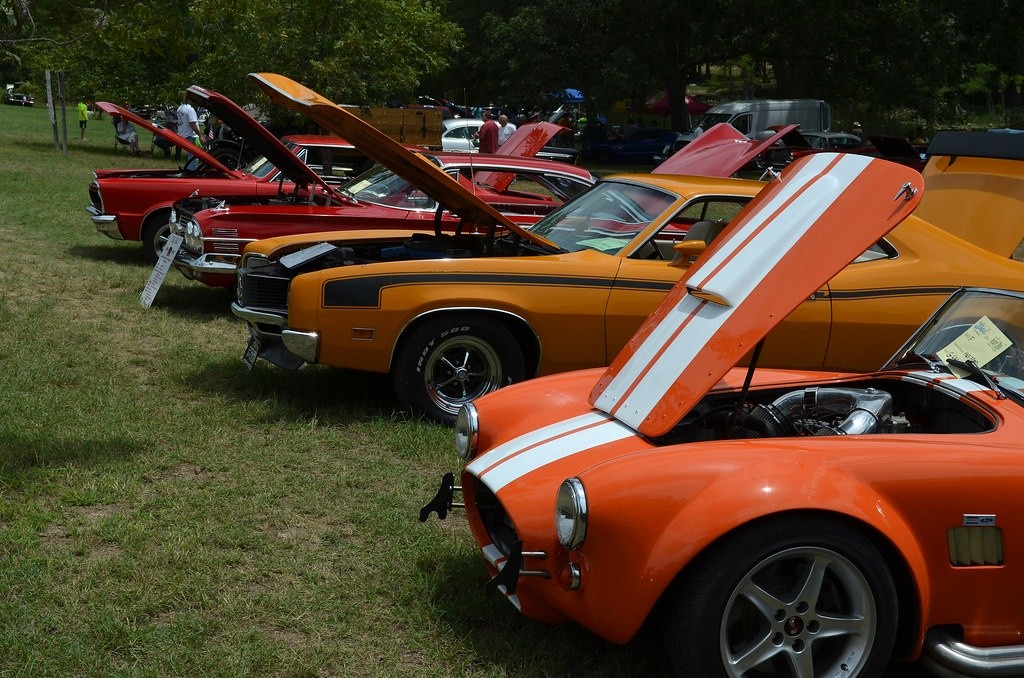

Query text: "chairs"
[113, 129, 135, 155]
[671, 220, 726, 262]
[150, 122, 178, 160]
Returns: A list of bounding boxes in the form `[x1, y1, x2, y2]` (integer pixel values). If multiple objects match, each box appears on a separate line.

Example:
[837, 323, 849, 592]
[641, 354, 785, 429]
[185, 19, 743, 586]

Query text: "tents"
[625, 83, 712, 132]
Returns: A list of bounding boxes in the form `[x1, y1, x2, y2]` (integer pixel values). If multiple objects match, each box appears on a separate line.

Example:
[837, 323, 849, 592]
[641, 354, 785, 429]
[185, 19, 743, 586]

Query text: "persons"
[112, 116, 122, 134]
[204, 118, 219, 139]
[905, 127, 930, 144]
[474, 111, 498, 154]
[498, 115, 516, 146]
[511, 105, 608, 148]
[117, 116, 138, 156]
[156, 115, 183, 161]
[77, 96, 88, 140]
[849, 122, 864, 139]
[624, 118, 658, 139]
[176, 92, 206, 170]
[388, 93, 510, 121]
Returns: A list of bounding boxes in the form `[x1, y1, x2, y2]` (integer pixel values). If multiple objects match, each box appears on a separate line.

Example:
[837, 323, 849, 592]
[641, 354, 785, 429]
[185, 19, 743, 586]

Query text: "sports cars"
[416, 150, 1024, 678]
[85, 71, 1024, 433]
